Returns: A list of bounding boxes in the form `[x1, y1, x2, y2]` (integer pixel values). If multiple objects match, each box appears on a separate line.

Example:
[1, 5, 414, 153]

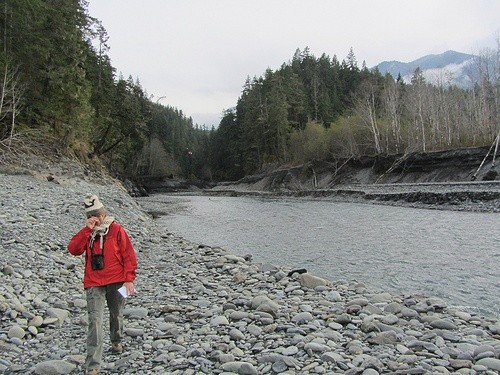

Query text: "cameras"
[91, 253, 104, 271]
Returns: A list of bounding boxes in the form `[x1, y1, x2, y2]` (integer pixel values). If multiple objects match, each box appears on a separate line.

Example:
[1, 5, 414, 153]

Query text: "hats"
[84, 192, 104, 217]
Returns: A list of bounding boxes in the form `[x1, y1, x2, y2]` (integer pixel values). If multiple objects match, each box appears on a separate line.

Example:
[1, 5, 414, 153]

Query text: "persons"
[67, 196, 138, 375]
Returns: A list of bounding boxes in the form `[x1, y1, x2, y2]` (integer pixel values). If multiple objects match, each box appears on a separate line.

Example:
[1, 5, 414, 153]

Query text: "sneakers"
[111, 342, 122, 355]
[86, 364, 101, 375]
